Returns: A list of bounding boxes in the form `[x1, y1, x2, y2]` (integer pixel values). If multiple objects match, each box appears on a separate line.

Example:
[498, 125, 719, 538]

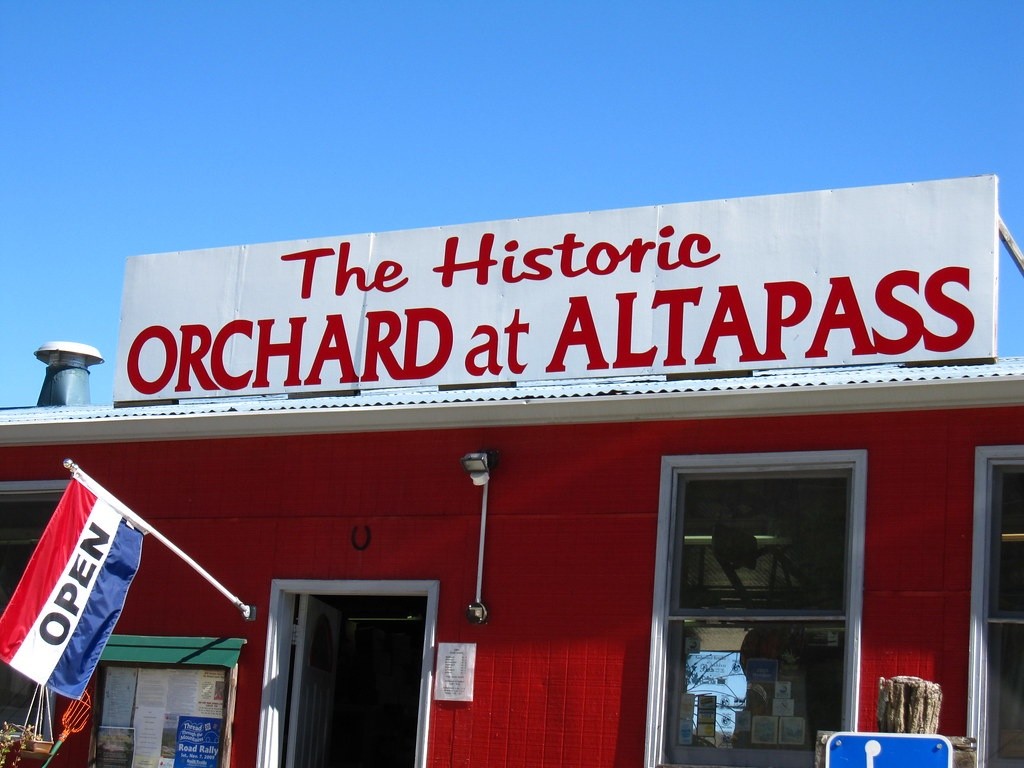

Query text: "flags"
[0, 469, 148, 700]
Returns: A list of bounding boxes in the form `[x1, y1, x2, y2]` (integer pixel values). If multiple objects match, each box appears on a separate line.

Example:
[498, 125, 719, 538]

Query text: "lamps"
[460, 448, 499, 474]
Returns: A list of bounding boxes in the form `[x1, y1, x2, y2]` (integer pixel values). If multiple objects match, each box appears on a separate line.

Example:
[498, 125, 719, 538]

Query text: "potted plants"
[0, 720, 54, 768]
